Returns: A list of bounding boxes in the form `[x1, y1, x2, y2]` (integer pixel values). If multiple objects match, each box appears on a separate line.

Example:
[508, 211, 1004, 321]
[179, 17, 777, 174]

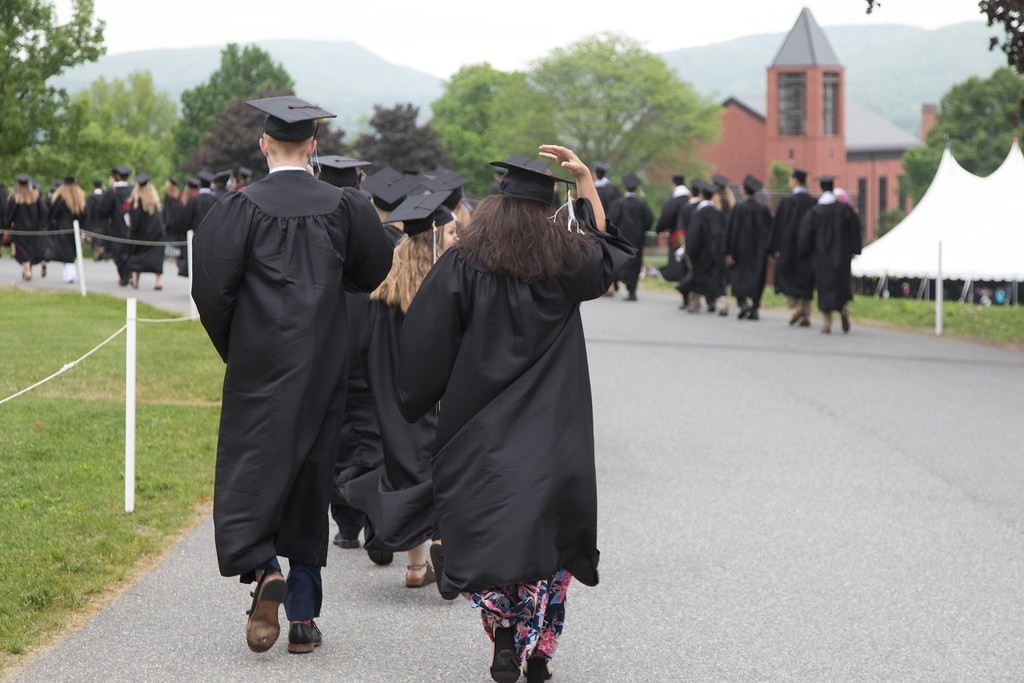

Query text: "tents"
[852, 137, 1024, 336]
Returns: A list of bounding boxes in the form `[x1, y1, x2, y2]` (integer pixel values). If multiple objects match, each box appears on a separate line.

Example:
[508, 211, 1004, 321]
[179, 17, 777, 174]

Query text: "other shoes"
[333, 533, 360, 549]
[365, 538, 394, 565]
[601, 283, 861, 338]
[1, 242, 185, 296]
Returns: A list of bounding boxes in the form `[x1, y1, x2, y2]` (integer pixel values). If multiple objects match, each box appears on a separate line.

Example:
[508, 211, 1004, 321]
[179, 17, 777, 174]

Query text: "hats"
[361, 165, 421, 211]
[308, 155, 374, 189]
[590, 157, 849, 198]
[0, 160, 262, 198]
[420, 171, 470, 199]
[380, 189, 456, 237]
[489, 159, 585, 238]
[245, 94, 338, 142]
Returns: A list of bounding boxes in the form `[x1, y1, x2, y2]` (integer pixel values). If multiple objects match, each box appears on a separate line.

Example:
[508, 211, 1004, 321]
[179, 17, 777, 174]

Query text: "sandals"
[427, 540, 459, 601]
[405, 561, 437, 588]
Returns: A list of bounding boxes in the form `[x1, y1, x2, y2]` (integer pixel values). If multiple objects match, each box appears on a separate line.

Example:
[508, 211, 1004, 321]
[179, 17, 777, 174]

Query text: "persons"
[657, 176, 690, 263]
[399, 143, 637, 683]
[607, 173, 654, 300]
[97, 165, 163, 289]
[165, 169, 246, 276]
[192, 98, 392, 653]
[0, 184, 8, 257]
[770, 169, 814, 326]
[673, 175, 741, 314]
[6, 175, 93, 283]
[797, 176, 861, 332]
[311, 154, 472, 585]
[725, 175, 773, 318]
[161, 177, 182, 223]
[88, 180, 106, 260]
[592, 162, 620, 296]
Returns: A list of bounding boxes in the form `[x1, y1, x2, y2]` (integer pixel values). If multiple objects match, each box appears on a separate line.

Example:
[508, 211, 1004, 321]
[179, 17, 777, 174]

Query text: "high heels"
[490, 628, 520, 683]
[526, 657, 553, 683]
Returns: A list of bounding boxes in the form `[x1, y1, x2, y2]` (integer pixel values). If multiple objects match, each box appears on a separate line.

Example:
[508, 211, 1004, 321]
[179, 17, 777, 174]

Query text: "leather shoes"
[288, 619, 324, 653]
[244, 569, 288, 653]
